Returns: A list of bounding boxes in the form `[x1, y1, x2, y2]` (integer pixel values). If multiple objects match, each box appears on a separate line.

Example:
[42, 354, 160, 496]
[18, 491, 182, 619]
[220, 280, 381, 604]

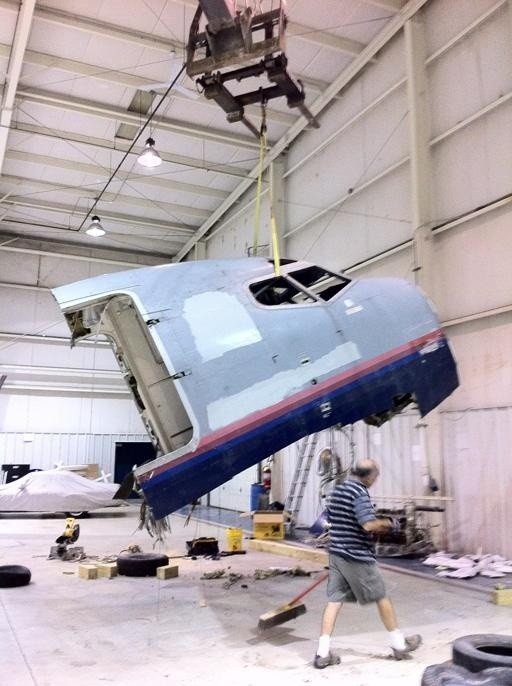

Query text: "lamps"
[87, 197, 105, 237]
[136, 89, 164, 168]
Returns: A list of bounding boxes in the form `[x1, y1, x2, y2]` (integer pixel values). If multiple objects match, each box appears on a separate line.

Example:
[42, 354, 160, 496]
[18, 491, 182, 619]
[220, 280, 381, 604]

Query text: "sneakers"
[314, 650, 340, 669]
[391, 634, 423, 661]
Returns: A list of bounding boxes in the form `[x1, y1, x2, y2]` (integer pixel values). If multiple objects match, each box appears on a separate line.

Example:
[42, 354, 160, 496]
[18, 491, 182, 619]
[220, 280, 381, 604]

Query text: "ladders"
[284, 433, 319, 537]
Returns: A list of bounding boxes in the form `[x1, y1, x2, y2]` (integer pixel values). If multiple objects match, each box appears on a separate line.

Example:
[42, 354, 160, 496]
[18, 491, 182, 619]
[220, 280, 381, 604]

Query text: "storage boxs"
[78, 561, 117, 579]
[154, 565, 178, 579]
[238, 508, 299, 540]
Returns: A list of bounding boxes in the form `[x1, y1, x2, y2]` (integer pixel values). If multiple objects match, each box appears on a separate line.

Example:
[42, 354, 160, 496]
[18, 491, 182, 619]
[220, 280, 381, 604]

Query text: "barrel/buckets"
[251, 484, 263, 519]
[227, 529, 243, 551]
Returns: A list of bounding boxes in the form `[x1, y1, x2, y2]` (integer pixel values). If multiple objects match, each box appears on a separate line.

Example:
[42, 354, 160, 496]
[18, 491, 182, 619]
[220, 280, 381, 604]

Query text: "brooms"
[258, 571, 335, 630]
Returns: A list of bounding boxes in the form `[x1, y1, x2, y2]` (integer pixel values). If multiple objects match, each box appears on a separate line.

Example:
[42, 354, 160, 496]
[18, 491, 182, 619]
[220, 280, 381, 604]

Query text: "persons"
[313, 457, 423, 669]
